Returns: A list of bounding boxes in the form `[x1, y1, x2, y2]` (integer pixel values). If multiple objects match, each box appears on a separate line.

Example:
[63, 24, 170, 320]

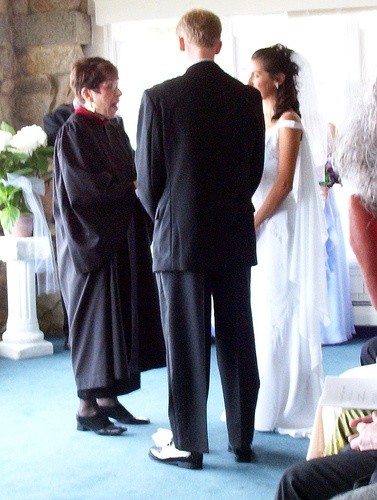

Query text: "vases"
[3, 214, 32, 236]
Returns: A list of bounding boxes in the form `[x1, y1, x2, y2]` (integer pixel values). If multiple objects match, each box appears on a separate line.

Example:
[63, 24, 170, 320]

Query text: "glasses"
[89, 81, 118, 91]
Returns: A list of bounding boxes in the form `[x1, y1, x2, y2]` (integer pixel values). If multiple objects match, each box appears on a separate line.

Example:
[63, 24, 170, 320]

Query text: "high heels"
[76, 408, 127, 435]
[98, 402, 150, 425]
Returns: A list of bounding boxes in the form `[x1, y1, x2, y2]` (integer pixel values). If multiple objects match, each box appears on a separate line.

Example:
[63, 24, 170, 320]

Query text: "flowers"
[0, 120, 55, 233]
[319, 161, 339, 200]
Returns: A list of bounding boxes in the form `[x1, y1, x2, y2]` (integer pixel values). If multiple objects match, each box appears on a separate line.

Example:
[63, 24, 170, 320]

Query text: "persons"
[50, 55, 151, 438]
[134, 9, 266, 467]
[242, 42, 311, 435]
[276, 73, 377, 500]
[299, 102, 343, 344]
[43, 59, 87, 147]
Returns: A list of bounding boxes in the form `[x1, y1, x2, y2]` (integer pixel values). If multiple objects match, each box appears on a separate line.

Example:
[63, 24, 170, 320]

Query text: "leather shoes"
[227, 441, 253, 463]
[149, 437, 203, 470]
